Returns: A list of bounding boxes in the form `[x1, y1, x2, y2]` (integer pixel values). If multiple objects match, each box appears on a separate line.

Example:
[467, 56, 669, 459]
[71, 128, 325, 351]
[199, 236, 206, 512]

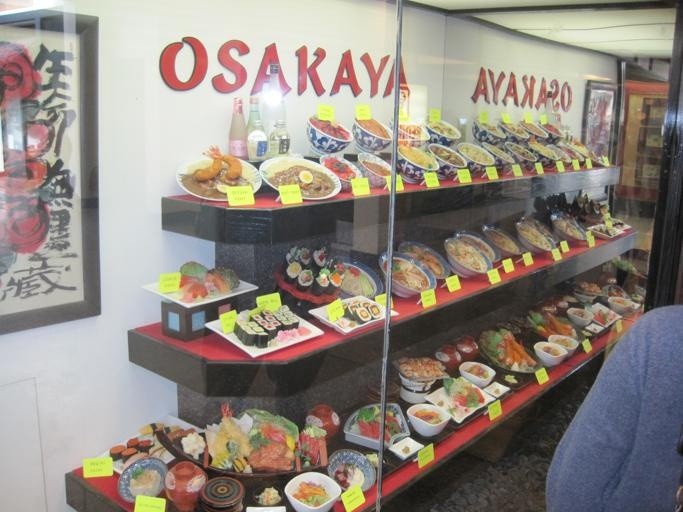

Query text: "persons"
[545, 303, 682, 512]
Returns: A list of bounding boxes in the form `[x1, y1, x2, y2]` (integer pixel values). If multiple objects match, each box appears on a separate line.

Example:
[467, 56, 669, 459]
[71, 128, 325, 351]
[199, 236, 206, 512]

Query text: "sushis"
[310, 249, 325, 273]
[324, 273, 342, 295]
[262, 316, 276, 322]
[256, 320, 268, 327]
[310, 273, 329, 296]
[345, 304, 360, 321]
[279, 305, 289, 314]
[348, 299, 361, 307]
[272, 311, 283, 318]
[237, 326, 251, 341]
[340, 302, 349, 318]
[353, 307, 371, 325]
[280, 320, 292, 332]
[296, 269, 314, 292]
[137, 440, 155, 455]
[365, 303, 382, 319]
[287, 316, 299, 330]
[109, 446, 125, 462]
[298, 247, 311, 270]
[251, 326, 265, 333]
[254, 332, 267, 348]
[202, 274, 231, 294]
[282, 261, 301, 284]
[283, 311, 293, 319]
[247, 321, 259, 328]
[179, 276, 199, 292]
[249, 314, 263, 323]
[263, 324, 277, 341]
[259, 310, 273, 319]
[153, 425, 179, 438]
[268, 320, 282, 337]
[233, 320, 247, 336]
[358, 300, 372, 310]
[139, 422, 164, 436]
[241, 330, 256, 346]
[127, 437, 140, 449]
[120, 448, 138, 464]
[275, 315, 287, 322]
[179, 283, 210, 303]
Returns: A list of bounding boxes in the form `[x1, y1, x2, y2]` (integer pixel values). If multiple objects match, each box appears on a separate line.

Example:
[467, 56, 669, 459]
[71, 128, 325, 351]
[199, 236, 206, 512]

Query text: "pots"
[399, 384, 431, 405]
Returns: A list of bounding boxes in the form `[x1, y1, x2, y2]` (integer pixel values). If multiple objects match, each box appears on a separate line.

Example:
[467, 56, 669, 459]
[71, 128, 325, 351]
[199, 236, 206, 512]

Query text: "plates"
[585, 322, 605, 333]
[308, 296, 399, 335]
[475, 329, 545, 373]
[141, 269, 259, 309]
[154, 427, 333, 479]
[603, 217, 632, 230]
[202, 304, 324, 359]
[259, 156, 342, 199]
[326, 448, 377, 493]
[588, 223, 625, 239]
[344, 403, 411, 452]
[117, 456, 168, 505]
[424, 376, 497, 425]
[585, 302, 622, 327]
[175, 154, 261, 203]
[97, 412, 205, 478]
[391, 356, 450, 381]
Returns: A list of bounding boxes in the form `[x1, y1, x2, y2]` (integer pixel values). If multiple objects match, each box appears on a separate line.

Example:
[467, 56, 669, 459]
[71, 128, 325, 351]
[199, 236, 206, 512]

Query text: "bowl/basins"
[471, 120, 506, 147]
[379, 251, 438, 299]
[524, 139, 557, 166]
[253, 483, 285, 507]
[538, 122, 563, 144]
[388, 118, 431, 145]
[356, 151, 392, 188]
[426, 119, 461, 145]
[497, 120, 529, 141]
[549, 212, 587, 242]
[444, 238, 493, 278]
[332, 257, 384, 301]
[459, 361, 496, 390]
[390, 145, 438, 185]
[482, 143, 515, 167]
[567, 307, 595, 326]
[454, 231, 502, 263]
[398, 241, 452, 280]
[481, 223, 521, 256]
[426, 143, 467, 180]
[483, 381, 511, 398]
[548, 335, 579, 359]
[284, 472, 342, 512]
[455, 142, 495, 174]
[556, 143, 584, 164]
[389, 437, 425, 461]
[405, 403, 452, 437]
[513, 220, 555, 254]
[572, 286, 600, 303]
[319, 153, 362, 191]
[521, 120, 548, 137]
[502, 140, 536, 171]
[548, 143, 571, 167]
[607, 296, 630, 315]
[305, 114, 352, 154]
[534, 341, 568, 367]
[352, 117, 391, 154]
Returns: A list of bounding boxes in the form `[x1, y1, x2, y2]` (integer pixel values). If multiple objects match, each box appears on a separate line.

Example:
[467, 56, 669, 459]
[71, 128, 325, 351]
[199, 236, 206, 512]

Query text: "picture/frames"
[1, 5, 102, 338]
[581, 80, 618, 164]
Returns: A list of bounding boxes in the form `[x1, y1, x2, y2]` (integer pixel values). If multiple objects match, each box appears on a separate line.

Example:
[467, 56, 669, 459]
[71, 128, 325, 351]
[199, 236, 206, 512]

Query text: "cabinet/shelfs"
[65, 145, 650, 512]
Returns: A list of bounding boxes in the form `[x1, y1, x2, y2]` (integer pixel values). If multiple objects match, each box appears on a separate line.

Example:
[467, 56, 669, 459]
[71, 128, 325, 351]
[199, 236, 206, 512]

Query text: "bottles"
[262, 64, 285, 137]
[244, 96, 268, 160]
[229, 97, 248, 161]
[268, 121, 290, 157]
